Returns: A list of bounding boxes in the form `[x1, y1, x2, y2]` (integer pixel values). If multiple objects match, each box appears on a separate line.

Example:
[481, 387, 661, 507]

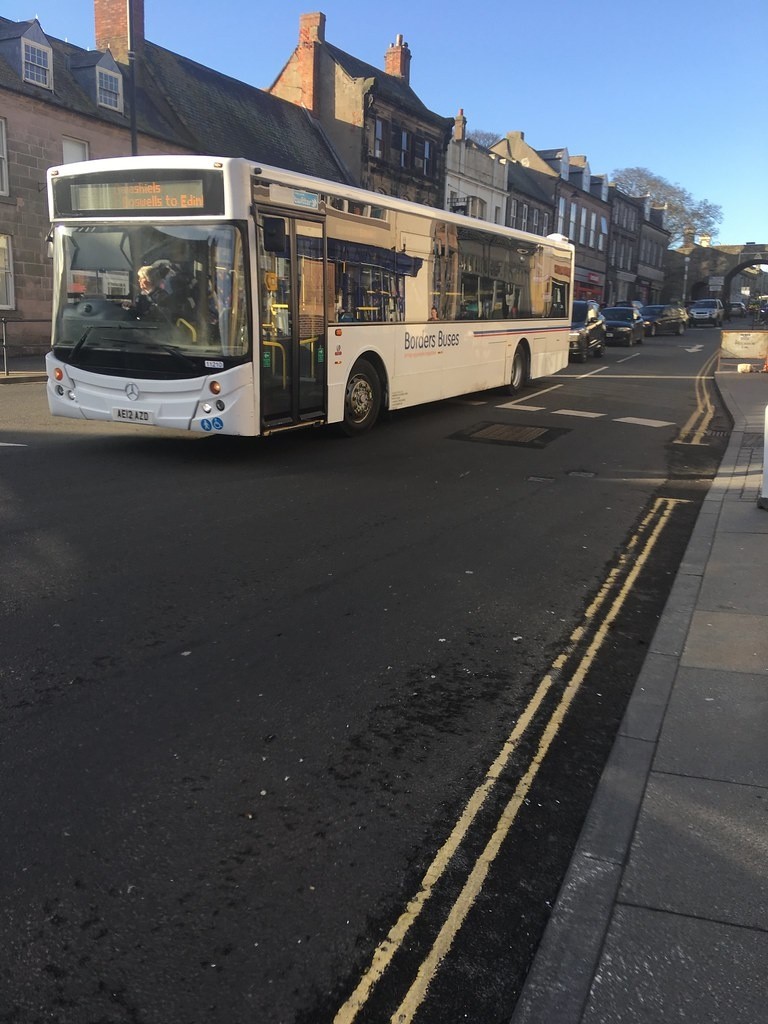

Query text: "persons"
[122, 266, 171, 322]
[722, 299, 732, 322]
[427, 307, 440, 320]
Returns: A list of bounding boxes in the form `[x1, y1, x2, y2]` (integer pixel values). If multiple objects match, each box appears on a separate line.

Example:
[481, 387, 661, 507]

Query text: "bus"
[43, 154, 575, 440]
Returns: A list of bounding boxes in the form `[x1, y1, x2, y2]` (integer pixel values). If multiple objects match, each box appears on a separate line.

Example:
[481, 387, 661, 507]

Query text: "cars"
[640, 305, 690, 336]
[569, 299, 607, 361]
[601, 307, 646, 346]
[689, 299, 724, 327]
[730, 302, 746, 318]
